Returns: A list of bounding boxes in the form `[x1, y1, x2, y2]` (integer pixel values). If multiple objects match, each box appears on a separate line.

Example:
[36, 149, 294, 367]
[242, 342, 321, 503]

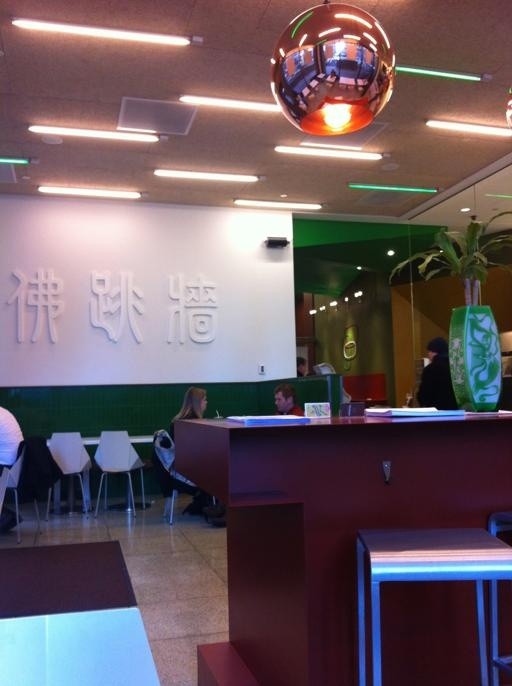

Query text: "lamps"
[273, 146, 384, 161]
[426, 119, 512, 137]
[233, 200, 325, 211]
[12, 17, 190, 48]
[0, 159, 32, 166]
[486, 194, 512, 200]
[27, 125, 160, 144]
[178, 94, 280, 114]
[347, 183, 439, 194]
[396, 67, 482, 83]
[36, 186, 143, 200]
[270, 3, 396, 137]
[152, 169, 261, 183]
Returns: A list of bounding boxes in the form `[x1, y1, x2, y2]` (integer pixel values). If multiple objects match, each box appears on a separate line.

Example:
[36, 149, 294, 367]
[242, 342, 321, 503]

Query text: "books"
[364, 406, 465, 418]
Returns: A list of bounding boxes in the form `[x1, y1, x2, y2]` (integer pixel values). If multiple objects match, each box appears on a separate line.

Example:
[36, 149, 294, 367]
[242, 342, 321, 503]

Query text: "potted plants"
[389, 210, 512, 412]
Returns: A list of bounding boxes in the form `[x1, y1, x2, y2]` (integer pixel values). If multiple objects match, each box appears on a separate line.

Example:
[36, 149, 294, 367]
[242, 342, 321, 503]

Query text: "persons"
[295, 357, 306, 375]
[274, 384, 304, 414]
[417, 339, 458, 410]
[168, 388, 210, 516]
[0, 404, 23, 534]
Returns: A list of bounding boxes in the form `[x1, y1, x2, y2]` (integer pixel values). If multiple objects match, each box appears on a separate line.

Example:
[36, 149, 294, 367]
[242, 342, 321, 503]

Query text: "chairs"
[154, 429, 216, 525]
[0, 437, 43, 544]
[46, 432, 92, 521]
[94, 431, 146, 518]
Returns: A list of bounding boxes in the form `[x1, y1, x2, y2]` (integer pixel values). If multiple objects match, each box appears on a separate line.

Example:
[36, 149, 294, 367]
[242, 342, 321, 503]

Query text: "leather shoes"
[0, 510, 23, 531]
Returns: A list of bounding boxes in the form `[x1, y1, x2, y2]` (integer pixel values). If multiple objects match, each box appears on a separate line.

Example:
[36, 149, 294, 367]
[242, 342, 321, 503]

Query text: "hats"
[426, 336, 448, 353]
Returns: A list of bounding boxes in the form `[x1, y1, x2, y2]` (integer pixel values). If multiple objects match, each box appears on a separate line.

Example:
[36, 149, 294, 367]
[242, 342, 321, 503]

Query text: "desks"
[174, 411, 512, 686]
[0, 541, 160, 686]
[46, 436, 154, 515]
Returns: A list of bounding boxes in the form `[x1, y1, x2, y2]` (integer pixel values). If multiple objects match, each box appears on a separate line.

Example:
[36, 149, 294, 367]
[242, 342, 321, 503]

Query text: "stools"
[356, 529, 512, 686]
